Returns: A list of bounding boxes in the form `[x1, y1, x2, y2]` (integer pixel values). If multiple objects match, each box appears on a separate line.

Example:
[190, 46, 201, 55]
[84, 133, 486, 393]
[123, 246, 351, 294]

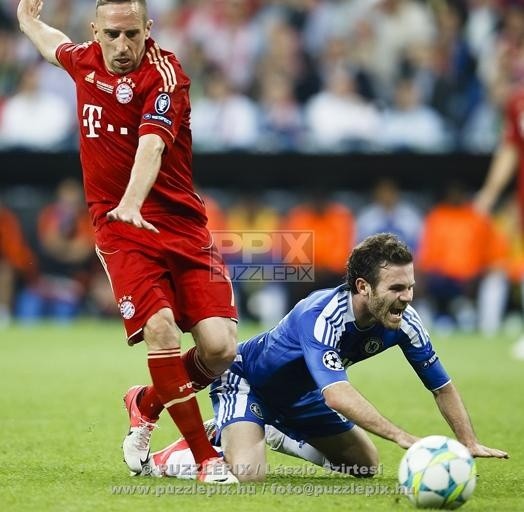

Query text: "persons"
[471, 85, 523, 364]
[147, 230, 510, 484]
[0, 0, 524, 334]
[14, 1, 238, 486]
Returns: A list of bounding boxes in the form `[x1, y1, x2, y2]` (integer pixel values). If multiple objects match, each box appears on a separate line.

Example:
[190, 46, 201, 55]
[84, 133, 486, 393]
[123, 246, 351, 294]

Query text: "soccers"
[399, 436, 476, 509]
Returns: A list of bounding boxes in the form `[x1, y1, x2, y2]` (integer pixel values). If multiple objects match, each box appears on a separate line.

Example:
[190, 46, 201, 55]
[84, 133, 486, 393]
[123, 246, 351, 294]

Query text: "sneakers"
[123, 384, 159, 475]
[194, 457, 239, 485]
[200, 418, 218, 441]
[130, 438, 193, 478]
